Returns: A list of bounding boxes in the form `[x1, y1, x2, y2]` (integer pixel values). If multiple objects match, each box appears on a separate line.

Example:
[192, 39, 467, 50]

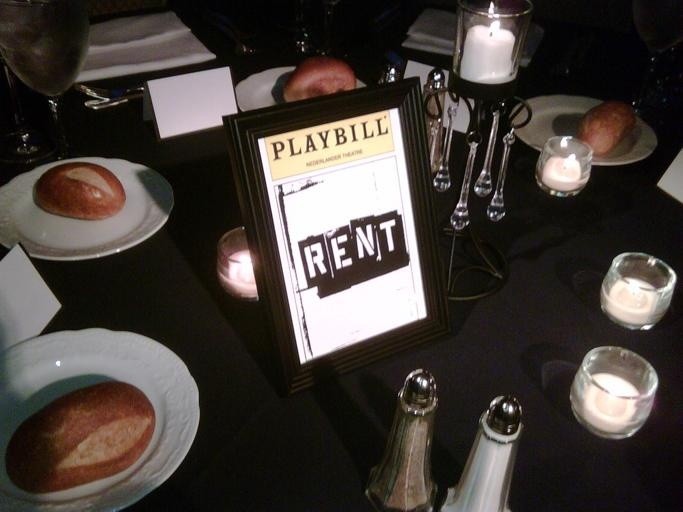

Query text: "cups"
[598, 250, 677, 334]
[213, 228, 257, 302]
[533, 136, 592, 200]
[569, 345, 661, 443]
[454, 0, 532, 84]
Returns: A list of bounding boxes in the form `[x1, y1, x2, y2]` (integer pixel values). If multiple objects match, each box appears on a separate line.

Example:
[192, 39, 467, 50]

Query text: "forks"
[69, 78, 145, 104]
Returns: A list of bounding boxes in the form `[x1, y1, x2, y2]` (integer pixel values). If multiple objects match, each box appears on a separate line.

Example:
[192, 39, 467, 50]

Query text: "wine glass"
[0, 0, 92, 166]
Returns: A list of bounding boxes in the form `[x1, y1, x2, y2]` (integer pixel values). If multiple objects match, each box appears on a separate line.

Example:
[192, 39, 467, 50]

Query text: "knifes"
[83, 97, 127, 109]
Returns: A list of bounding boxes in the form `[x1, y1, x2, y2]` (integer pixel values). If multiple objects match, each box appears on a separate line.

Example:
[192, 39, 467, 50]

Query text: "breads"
[32, 162, 127, 220]
[283, 56, 357, 104]
[579, 100, 636, 158]
[5, 381, 156, 493]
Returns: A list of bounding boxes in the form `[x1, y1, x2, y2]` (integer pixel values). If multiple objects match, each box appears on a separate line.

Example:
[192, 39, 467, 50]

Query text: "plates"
[510, 93, 660, 164]
[233, 65, 366, 116]
[1, 327, 202, 512]
[0, 156, 178, 261]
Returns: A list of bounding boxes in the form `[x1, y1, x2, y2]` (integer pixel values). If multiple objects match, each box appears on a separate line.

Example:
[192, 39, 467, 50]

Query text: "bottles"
[378, 61, 447, 174]
[365, 366, 521, 512]
